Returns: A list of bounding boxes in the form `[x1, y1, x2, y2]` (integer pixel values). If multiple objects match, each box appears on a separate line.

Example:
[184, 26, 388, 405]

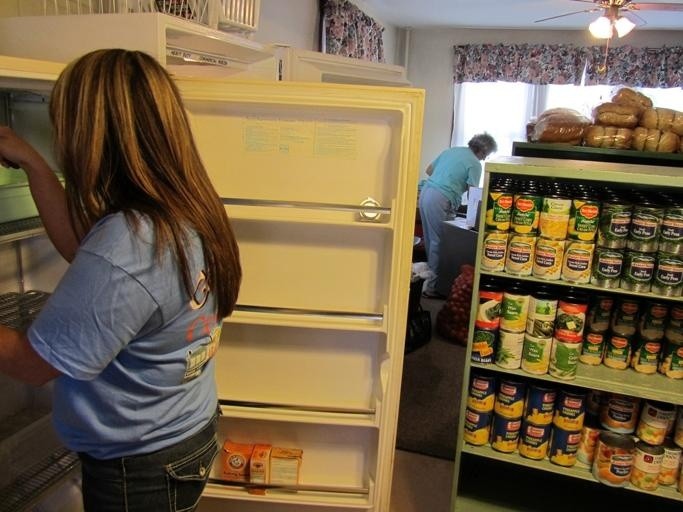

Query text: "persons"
[0, 46, 240, 510]
[420, 131, 498, 300]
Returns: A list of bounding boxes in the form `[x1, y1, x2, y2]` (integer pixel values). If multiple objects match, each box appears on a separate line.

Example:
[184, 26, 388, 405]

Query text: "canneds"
[463, 173, 683, 495]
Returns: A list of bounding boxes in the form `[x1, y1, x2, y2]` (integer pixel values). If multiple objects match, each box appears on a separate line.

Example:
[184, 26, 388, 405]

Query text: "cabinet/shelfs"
[445, 153, 682, 512]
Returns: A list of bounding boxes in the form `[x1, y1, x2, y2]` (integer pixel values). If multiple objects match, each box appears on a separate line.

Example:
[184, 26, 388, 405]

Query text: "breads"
[532, 88, 683, 153]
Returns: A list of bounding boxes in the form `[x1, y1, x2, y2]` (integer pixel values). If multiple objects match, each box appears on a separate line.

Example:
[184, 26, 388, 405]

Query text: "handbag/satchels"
[405, 303, 432, 353]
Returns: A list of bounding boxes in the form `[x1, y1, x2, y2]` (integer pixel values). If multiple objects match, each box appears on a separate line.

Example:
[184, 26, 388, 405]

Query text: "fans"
[531, 0, 682, 38]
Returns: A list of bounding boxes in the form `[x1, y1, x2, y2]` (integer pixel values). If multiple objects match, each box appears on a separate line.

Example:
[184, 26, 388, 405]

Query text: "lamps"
[587, 0, 636, 40]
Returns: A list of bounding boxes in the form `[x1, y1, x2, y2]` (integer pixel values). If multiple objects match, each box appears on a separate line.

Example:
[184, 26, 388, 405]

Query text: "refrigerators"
[0, 13, 415, 89]
[0, 48, 430, 511]
